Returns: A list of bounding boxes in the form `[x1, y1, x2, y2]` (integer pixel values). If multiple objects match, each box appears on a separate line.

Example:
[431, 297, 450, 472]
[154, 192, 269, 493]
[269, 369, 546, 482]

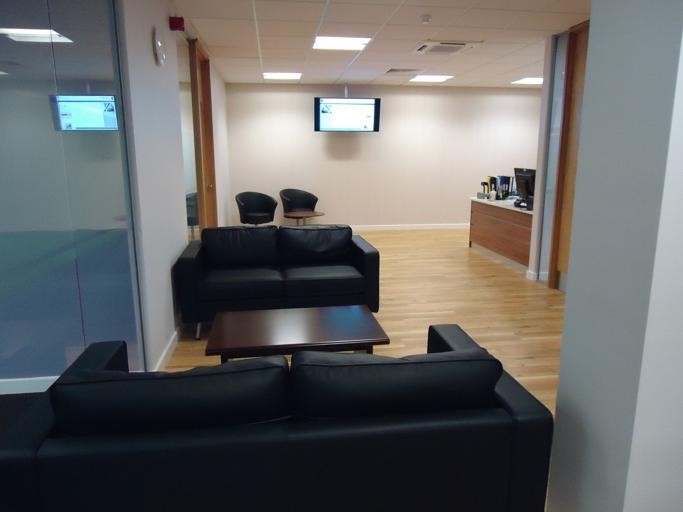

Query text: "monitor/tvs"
[47, 92, 123, 132]
[313, 97, 381, 133]
[513, 167, 536, 211]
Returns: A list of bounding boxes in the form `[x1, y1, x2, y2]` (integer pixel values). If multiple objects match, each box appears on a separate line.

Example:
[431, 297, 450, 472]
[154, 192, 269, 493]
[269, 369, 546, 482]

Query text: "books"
[480, 174, 515, 199]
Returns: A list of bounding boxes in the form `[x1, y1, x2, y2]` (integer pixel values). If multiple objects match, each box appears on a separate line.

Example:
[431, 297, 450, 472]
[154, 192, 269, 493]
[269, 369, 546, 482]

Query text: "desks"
[467, 195, 532, 268]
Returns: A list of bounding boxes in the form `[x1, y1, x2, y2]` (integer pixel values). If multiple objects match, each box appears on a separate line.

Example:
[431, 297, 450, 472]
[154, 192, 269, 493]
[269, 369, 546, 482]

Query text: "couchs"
[170, 223, 380, 340]
[1, 324, 554, 512]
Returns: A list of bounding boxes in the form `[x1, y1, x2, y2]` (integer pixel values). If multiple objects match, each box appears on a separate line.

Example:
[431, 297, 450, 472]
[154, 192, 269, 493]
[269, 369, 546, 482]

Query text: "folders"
[498, 176, 510, 197]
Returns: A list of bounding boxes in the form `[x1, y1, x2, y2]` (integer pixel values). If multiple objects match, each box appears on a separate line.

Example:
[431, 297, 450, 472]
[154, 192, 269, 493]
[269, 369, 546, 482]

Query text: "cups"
[489, 191, 496, 202]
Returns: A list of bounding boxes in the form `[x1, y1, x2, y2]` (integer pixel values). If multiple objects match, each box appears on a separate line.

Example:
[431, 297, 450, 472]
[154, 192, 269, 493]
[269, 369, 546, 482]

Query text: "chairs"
[235, 188, 318, 226]
[183, 191, 197, 242]
